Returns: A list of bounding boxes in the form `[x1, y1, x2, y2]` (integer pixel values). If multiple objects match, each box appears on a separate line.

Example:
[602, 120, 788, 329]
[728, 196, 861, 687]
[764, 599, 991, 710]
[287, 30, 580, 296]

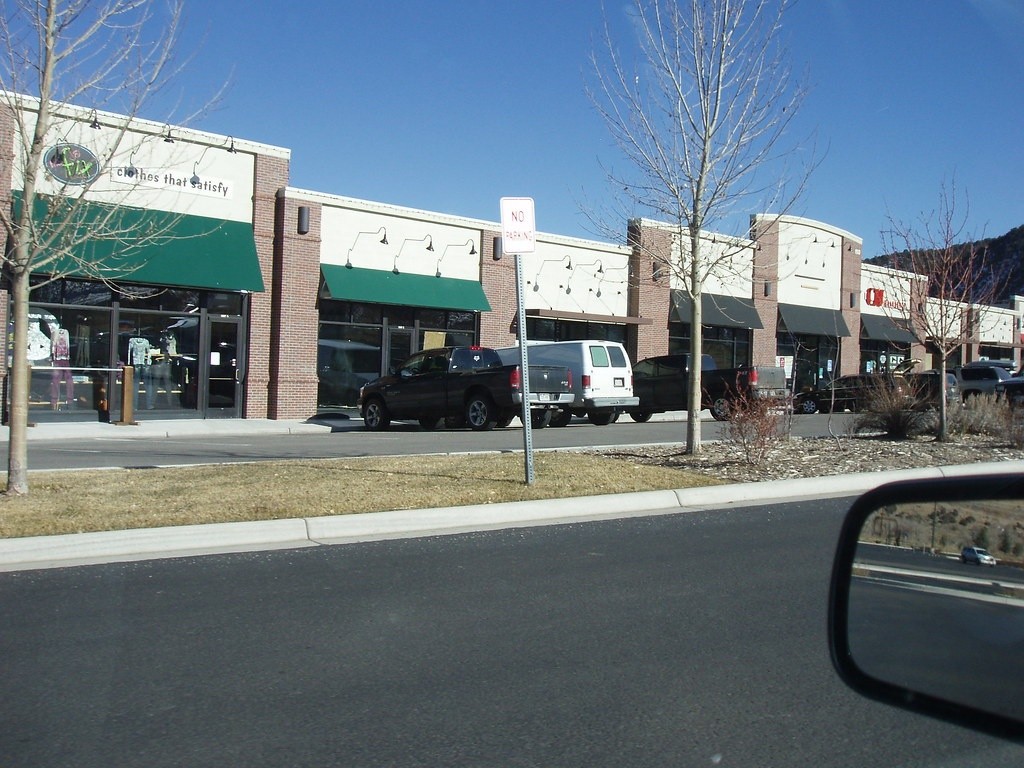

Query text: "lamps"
[887, 270, 922, 283]
[360, 227, 389, 245]
[83, 109, 102, 130]
[705, 231, 763, 252]
[219, 135, 237, 154]
[297, 206, 310, 236]
[803, 233, 819, 244]
[578, 259, 606, 274]
[160, 124, 174, 143]
[822, 238, 836, 248]
[405, 234, 435, 253]
[448, 239, 478, 255]
[605, 263, 634, 279]
[947, 314, 951, 318]
[842, 241, 853, 252]
[543, 255, 575, 272]
[1003, 320, 1007, 325]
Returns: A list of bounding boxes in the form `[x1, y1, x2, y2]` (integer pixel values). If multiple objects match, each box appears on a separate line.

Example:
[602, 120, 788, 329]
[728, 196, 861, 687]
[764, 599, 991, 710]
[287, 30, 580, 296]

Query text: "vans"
[493, 340, 641, 428]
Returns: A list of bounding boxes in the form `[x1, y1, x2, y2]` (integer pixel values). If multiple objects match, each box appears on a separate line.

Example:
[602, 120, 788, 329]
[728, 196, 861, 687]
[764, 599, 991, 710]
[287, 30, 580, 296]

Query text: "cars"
[317, 340, 380, 398]
[902, 372, 963, 408]
[961, 546, 995, 566]
[947, 359, 1024, 409]
[791, 358, 923, 416]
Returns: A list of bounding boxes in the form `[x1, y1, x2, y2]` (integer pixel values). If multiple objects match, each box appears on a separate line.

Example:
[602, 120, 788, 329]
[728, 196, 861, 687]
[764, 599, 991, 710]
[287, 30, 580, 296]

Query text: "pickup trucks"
[359, 345, 575, 431]
[630, 354, 791, 421]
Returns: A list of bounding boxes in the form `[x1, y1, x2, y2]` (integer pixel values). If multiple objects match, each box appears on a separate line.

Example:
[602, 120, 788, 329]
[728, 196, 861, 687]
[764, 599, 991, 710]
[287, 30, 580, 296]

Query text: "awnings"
[859, 314, 919, 343]
[671, 289, 764, 330]
[10, 189, 267, 294]
[319, 263, 493, 312]
[777, 303, 852, 337]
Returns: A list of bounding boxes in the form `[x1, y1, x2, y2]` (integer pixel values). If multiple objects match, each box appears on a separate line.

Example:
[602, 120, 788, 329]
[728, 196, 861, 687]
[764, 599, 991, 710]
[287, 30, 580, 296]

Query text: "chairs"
[420, 356, 447, 373]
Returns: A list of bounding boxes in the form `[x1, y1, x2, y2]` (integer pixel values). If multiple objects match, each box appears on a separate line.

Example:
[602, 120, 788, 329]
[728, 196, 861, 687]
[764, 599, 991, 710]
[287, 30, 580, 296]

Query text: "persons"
[127, 337, 154, 408]
[50, 329, 76, 409]
